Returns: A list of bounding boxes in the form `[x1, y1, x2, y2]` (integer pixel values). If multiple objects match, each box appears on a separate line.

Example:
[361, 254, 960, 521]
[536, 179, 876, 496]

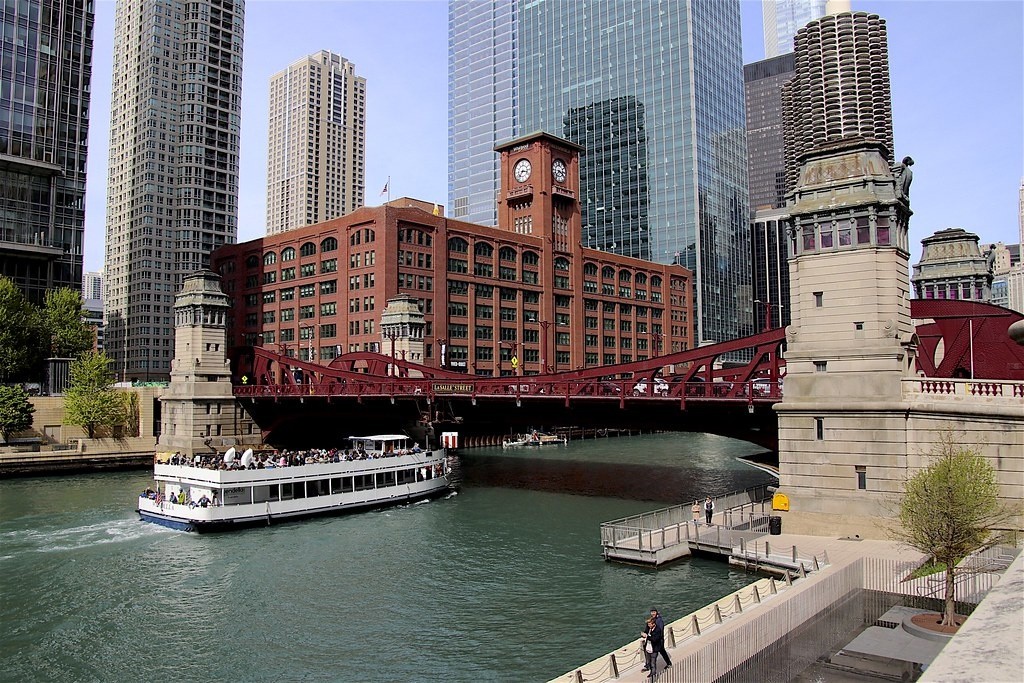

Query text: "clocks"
[512, 158, 533, 183]
[551, 158, 568, 184]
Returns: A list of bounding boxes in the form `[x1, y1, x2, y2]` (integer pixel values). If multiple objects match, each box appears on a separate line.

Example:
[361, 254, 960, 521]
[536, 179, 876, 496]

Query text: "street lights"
[376, 331, 411, 396]
[241, 333, 264, 385]
[754, 300, 783, 330]
[529, 318, 566, 374]
[269, 342, 304, 395]
[297, 322, 321, 395]
[641, 331, 667, 357]
[497, 340, 525, 375]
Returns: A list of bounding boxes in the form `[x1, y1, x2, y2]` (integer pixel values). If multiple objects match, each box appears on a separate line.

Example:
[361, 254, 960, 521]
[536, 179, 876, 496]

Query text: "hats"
[650, 606, 657, 612]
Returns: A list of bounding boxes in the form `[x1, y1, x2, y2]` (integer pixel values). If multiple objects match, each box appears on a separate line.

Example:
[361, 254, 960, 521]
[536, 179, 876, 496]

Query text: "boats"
[136, 433, 454, 535]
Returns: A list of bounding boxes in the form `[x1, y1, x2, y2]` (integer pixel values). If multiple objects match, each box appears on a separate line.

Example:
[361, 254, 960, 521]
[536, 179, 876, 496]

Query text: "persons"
[143, 486, 222, 508]
[691, 500, 701, 527]
[641, 618, 661, 679]
[156, 442, 422, 472]
[416, 464, 444, 483]
[642, 607, 672, 669]
[704, 495, 715, 525]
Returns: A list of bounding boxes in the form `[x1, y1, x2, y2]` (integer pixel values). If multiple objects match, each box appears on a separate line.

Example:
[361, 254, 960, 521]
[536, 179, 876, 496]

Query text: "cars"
[667, 376, 732, 397]
[632, 377, 670, 397]
[736, 377, 783, 399]
[576, 381, 627, 397]
[508, 384, 545, 394]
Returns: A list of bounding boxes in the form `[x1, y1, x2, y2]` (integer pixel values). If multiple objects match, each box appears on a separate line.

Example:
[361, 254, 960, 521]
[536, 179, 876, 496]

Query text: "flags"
[382, 182, 388, 193]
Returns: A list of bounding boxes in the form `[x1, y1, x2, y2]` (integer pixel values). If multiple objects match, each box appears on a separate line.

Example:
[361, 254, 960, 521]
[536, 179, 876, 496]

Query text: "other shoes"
[647, 672, 656, 678]
[663, 664, 672, 670]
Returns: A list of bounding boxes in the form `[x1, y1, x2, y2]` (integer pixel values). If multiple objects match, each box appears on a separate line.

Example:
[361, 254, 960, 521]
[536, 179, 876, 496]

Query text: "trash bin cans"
[769, 516, 781, 535]
